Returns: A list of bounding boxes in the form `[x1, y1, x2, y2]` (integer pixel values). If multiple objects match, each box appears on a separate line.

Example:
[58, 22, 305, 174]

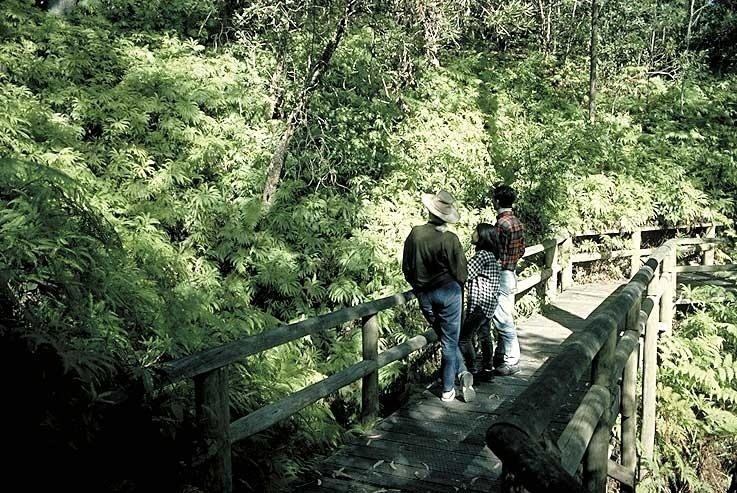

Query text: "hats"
[421, 189, 460, 223]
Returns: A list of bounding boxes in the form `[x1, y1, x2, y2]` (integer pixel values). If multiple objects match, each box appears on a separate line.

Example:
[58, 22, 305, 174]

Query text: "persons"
[493, 184, 525, 375]
[460, 222, 501, 383]
[402, 191, 476, 402]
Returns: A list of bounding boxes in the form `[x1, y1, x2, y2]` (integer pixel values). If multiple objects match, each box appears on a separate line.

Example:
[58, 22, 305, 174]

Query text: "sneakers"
[441, 385, 460, 401]
[496, 362, 520, 374]
[460, 371, 476, 402]
[468, 367, 478, 373]
